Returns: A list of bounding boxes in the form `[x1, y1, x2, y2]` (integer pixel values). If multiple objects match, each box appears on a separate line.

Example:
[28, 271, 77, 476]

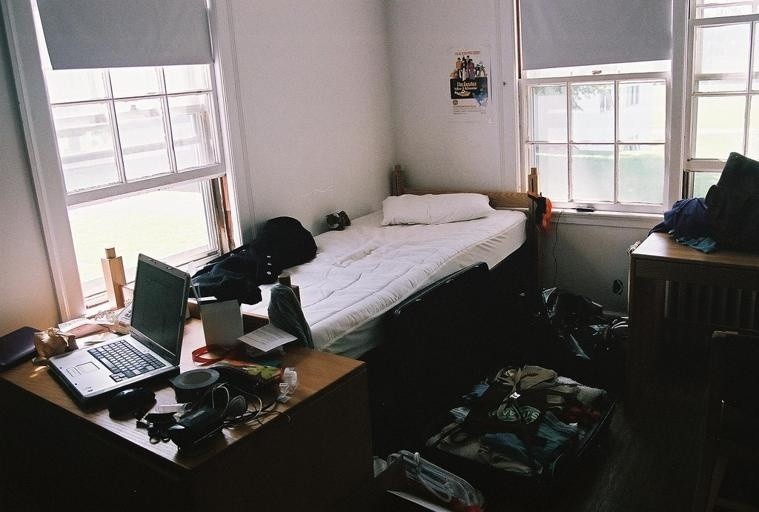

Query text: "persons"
[456, 55, 476, 80]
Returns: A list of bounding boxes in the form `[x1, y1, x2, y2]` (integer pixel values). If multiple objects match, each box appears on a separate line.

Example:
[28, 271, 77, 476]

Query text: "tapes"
[172, 369, 220, 403]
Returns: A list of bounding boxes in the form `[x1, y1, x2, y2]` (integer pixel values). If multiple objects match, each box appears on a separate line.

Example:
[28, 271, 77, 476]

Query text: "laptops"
[48, 253, 191, 405]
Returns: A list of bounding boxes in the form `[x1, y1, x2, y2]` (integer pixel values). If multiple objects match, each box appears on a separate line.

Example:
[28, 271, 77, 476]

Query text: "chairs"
[691, 334, 759, 512]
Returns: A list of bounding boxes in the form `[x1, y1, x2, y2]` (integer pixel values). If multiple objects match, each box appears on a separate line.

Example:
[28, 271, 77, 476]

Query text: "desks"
[3, 304, 373, 511]
[624, 230, 759, 411]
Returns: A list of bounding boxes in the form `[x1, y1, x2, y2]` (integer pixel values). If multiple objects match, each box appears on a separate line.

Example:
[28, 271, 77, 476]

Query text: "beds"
[98, 164, 541, 361]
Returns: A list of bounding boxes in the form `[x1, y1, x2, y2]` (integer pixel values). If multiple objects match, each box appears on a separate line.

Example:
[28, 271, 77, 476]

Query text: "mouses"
[108, 387, 155, 412]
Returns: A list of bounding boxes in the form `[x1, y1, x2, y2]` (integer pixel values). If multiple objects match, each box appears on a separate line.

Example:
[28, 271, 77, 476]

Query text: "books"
[0, 326, 43, 368]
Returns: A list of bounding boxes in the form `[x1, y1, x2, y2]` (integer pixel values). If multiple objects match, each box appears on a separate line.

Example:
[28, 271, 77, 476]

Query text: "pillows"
[376, 192, 496, 228]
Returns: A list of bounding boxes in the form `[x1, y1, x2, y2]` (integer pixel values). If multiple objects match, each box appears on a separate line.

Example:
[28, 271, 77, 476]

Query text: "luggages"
[386, 262, 617, 490]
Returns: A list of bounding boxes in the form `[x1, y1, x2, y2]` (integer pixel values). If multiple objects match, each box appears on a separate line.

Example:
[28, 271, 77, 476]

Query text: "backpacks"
[188, 216, 317, 304]
[653, 152, 758, 250]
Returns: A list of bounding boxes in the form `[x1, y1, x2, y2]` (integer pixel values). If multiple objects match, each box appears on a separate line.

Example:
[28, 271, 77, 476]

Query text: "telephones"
[115, 302, 133, 335]
